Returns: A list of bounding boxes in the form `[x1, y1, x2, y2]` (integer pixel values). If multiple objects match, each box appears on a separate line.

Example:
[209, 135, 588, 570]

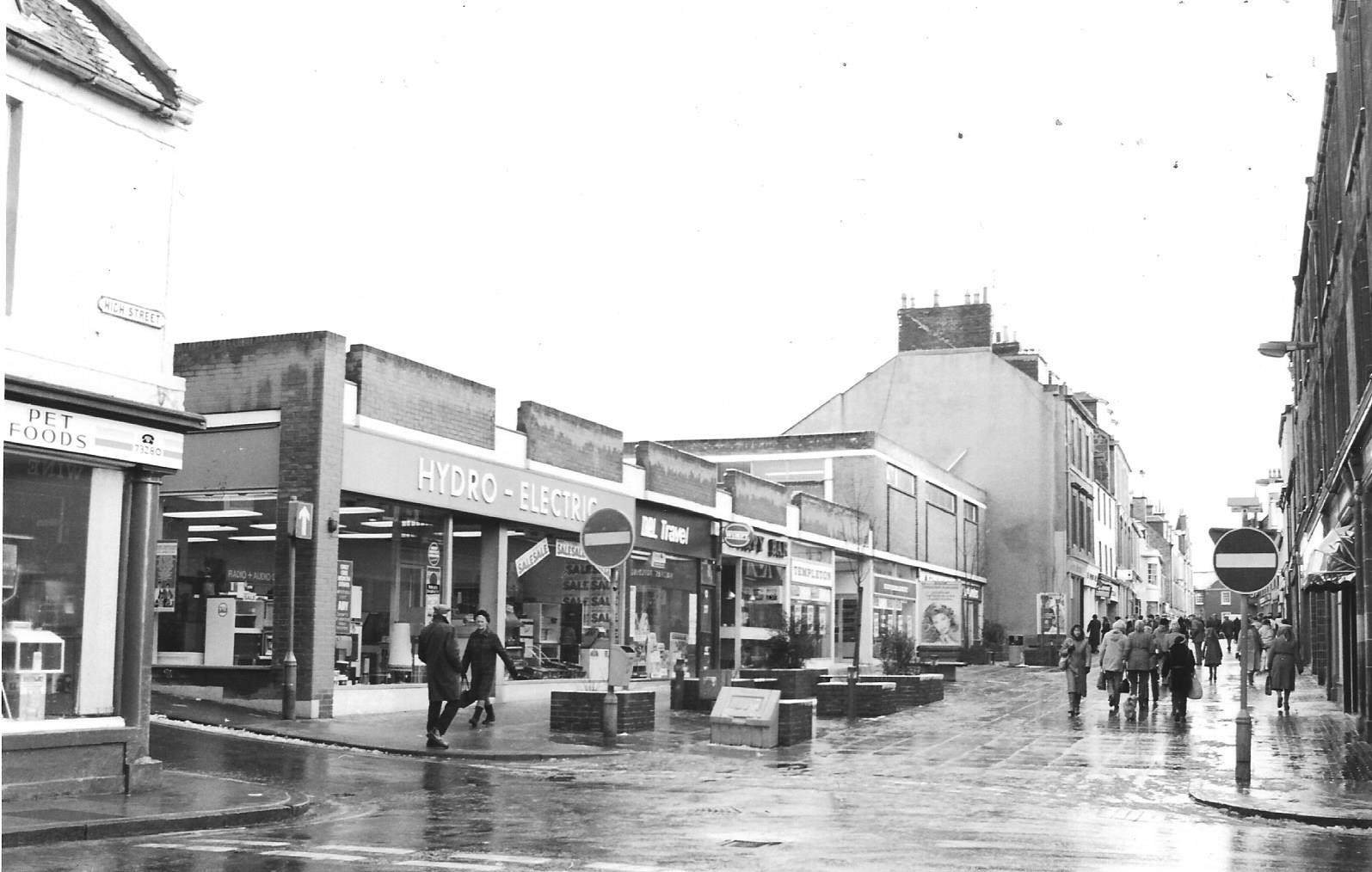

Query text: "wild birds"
[1265, 72, 1274, 79]
[1286, 92, 1296, 102]
[1282, 0, 1290, 4]
[1054, 118, 1063, 127]
[1172, 160, 1180, 170]
[957, 132, 964, 139]
[1243, 0, 1249, 4]
[1178, 0, 1183, 4]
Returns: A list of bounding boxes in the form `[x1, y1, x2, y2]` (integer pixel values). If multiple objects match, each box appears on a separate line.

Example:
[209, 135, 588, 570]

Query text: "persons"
[1086, 613, 1222, 722]
[1222, 611, 1304, 711]
[418, 604, 466, 748]
[461, 610, 522, 728]
[922, 603, 959, 646]
[1058, 625, 1092, 717]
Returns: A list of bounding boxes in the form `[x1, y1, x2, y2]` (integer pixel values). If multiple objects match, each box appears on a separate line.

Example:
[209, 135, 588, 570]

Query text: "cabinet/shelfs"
[520, 600, 563, 664]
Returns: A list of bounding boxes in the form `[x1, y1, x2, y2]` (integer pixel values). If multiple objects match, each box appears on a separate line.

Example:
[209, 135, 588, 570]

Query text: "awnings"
[1299, 524, 1358, 591]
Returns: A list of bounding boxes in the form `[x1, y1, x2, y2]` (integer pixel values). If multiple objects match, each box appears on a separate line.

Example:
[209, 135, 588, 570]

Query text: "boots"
[1074, 695, 1081, 714]
[468, 705, 483, 727]
[1067, 692, 1076, 713]
[482, 704, 495, 724]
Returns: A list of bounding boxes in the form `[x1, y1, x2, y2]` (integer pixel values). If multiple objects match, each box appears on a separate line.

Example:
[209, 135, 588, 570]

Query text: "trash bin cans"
[608, 645, 637, 688]
[1008, 634, 1024, 665]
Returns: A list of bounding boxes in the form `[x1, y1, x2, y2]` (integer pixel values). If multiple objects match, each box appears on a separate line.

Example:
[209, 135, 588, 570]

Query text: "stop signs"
[580, 507, 634, 569]
[1211, 526, 1280, 595]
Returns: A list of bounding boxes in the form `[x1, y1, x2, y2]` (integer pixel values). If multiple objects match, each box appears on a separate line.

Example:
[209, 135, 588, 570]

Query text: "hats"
[434, 604, 450, 614]
[473, 608, 490, 623]
[1160, 618, 1172, 624]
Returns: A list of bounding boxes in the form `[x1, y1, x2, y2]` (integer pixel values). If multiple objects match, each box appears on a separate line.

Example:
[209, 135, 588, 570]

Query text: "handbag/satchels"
[1121, 679, 1130, 693]
[1188, 673, 1202, 699]
[1058, 657, 1067, 669]
[1096, 668, 1106, 690]
[457, 689, 475, 708]
[1265, 676, 1272, 696]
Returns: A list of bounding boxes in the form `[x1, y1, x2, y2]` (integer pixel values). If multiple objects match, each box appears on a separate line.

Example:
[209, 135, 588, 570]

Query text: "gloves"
[508, 664, 518, 679]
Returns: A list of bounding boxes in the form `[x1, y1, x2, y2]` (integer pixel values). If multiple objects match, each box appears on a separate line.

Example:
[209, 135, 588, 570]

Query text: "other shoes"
[426, 728, 450, 749]
[1179, 717, 1185, 723]
[427, 738, 441, 746]
[1277, 697, 1282, 708]
[1108, 695, 1120, 709]
[1284, 703, 1290, 710]
